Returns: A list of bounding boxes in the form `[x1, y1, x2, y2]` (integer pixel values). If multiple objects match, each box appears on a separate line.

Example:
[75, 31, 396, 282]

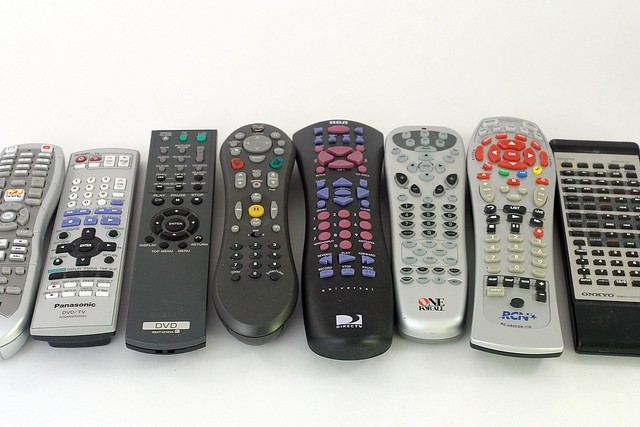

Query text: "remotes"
[29, 147, 140, 347]
[467, 116, 564, 358]
[384, 125, 469, 345]
[0, 143, 65, 360]
[122, 128, 220, 355]
[212, 123, 299, 346]
[549, 139, 640, 357]
[292, 118, 394, 360]
